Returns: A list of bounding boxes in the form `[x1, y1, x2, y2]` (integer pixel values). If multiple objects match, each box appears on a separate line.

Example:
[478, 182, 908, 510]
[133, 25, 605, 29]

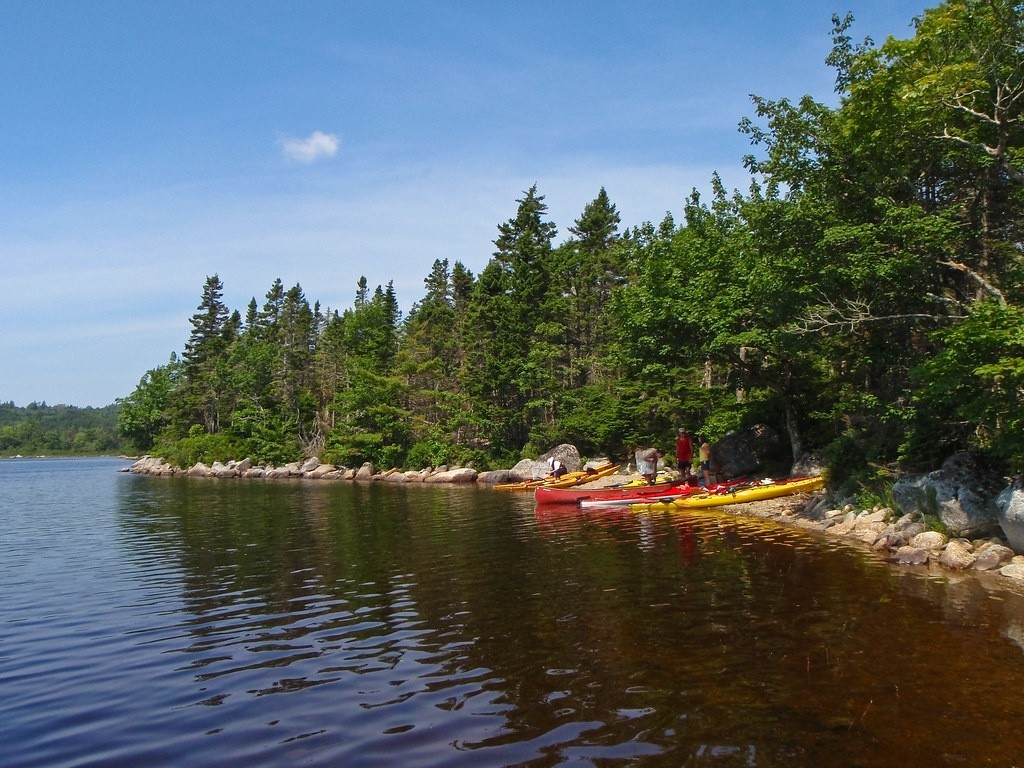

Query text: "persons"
[634, 439, 658, 485]
[545, 457, 568, 480]
[696, 434, 712, 486]
[675, 428, 694, 479]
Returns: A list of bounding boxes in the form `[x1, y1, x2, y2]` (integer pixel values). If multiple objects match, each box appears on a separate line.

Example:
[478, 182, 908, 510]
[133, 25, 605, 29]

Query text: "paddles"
[576, 468, 598, 479]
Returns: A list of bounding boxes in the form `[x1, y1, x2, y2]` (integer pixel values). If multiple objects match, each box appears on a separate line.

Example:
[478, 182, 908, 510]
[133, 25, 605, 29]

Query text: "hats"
[547, 457, 553, 467]
[679, 428, 686, 433]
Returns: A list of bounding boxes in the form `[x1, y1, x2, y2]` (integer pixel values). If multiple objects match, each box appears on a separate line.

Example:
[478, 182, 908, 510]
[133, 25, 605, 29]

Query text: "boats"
[491, 462, 828, 517]
